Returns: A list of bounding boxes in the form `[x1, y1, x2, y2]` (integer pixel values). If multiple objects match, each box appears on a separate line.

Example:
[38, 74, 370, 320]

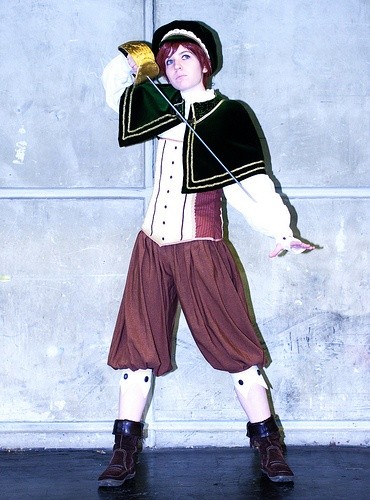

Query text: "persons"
[95, 19, 316, 486]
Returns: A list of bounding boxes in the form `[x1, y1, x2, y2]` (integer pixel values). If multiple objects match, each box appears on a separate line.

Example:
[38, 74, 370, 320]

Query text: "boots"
[245, 414, 298, 487]
[96, 419, 145, 488]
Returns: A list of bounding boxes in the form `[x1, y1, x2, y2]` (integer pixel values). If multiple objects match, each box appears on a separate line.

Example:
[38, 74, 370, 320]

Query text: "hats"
[151, 20, 225, 80]
